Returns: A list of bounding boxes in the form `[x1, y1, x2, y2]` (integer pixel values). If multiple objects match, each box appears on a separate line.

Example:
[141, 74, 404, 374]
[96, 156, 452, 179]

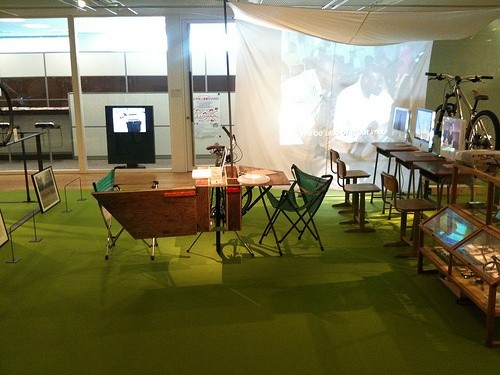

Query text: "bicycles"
[425, 69, 500, 169]
[208, 125, 253, 220]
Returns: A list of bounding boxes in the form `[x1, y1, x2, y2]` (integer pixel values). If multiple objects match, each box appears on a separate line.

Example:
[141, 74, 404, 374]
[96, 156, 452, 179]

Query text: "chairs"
[92, 166, 155, 259]
[258, 164, 333, 252]
[329, 149, 380, 234]
[380, 172, 439, 259]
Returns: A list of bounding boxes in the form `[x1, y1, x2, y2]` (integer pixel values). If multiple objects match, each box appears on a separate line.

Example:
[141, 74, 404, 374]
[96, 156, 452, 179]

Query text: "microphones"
[221, 126, 234, 139]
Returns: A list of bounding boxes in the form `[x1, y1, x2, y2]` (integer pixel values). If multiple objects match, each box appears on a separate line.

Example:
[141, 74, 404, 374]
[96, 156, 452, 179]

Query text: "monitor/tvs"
[441, 116, 467, 168]
[392, 106, 411, 146]
[112, 107, 146, 133]
[413, 107, 438, 156]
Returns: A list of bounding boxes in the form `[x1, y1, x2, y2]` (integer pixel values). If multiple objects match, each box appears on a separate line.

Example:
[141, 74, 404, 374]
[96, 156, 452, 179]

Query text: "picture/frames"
[0, 209, 9, 249]
[31, 166, 61, 214]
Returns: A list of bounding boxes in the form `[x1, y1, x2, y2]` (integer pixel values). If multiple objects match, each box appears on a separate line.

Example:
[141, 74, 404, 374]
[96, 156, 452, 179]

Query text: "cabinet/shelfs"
[419, 163, 500, 348]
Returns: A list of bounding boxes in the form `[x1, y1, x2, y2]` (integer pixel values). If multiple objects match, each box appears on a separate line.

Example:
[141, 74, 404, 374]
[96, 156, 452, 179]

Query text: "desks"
[369, 141, 471, 244]
[195, 166, 290, 259]
